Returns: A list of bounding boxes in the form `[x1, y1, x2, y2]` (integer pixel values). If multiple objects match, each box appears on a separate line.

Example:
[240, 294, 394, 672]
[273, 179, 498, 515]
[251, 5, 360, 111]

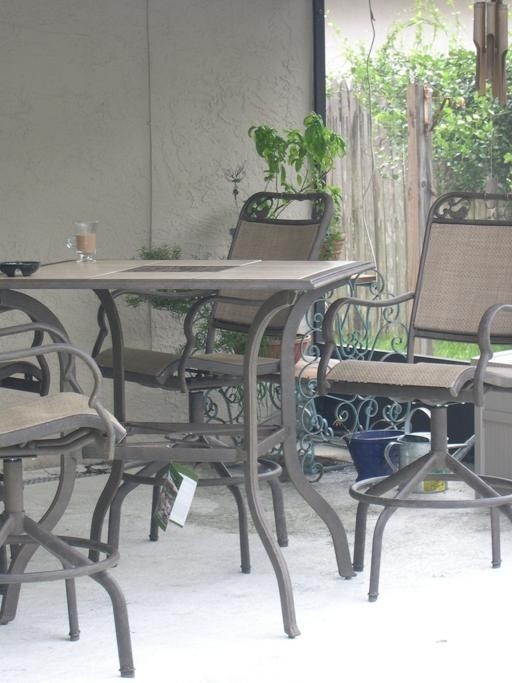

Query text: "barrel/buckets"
[343, 430, 405, 476]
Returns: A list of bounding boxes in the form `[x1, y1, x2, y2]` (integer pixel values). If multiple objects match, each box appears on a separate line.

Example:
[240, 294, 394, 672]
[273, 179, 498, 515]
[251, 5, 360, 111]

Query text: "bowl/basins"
[0, 260, 41, 277]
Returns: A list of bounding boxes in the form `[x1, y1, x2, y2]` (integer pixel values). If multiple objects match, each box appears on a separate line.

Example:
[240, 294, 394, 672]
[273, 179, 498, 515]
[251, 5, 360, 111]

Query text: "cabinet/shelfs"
[470, 350, 511, 499]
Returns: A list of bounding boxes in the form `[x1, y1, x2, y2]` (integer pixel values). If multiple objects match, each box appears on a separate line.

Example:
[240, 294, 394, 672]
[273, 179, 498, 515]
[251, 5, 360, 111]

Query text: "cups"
[63, 220, 100, 263]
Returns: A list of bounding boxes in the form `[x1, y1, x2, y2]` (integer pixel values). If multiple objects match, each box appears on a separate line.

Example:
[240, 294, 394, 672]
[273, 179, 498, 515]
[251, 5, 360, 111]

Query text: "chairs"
[0, 193, 376, 679]
[317, 192, 511, 603]
